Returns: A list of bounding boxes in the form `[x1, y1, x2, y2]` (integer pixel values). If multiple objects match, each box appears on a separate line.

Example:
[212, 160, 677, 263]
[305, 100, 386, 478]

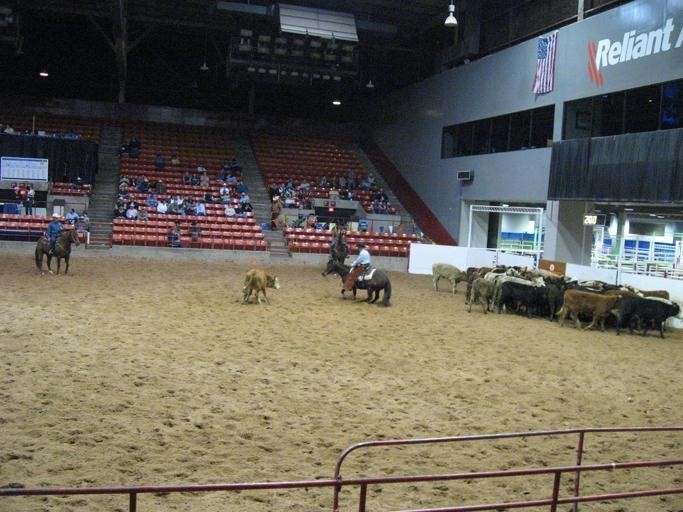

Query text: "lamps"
[333, 100, 342, 106]
[444, 0, 458, 27]
[201, 63, 208, 70]
[40, 69, 49, 77]
[366, 80, 375, 88]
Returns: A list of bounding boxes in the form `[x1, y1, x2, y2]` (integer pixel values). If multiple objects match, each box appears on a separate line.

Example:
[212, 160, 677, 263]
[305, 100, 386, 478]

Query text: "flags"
[529, 31, 557, 94]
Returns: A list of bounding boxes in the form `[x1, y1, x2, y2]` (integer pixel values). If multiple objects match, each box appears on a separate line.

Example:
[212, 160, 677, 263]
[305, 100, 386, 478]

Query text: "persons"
[350, 243, 372, 275]
[65, 208, 89, 233]
[112, 136, 254, 247]
[47, 212, 63, 257]
[12, 182, 34, 214]
[269, 172, 390, 230]
[328, 223, 350, 258]
[69, 176, 86, 190]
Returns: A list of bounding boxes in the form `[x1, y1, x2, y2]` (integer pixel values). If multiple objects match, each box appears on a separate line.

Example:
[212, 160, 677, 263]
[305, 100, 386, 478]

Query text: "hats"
[355, 242, 366, 248]
[51, 213, 61, 218]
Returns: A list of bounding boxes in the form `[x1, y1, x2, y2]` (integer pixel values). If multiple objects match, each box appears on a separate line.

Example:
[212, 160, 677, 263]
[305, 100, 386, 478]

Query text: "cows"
[465, 265, 681, 339]
[240, 268, 281, 306]
[431, 262, 468, 295]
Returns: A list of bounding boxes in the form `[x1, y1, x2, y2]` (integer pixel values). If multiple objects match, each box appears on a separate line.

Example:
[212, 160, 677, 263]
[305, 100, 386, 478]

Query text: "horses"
[34, 228, 81, 276]
[321, 259, 391, 307]
